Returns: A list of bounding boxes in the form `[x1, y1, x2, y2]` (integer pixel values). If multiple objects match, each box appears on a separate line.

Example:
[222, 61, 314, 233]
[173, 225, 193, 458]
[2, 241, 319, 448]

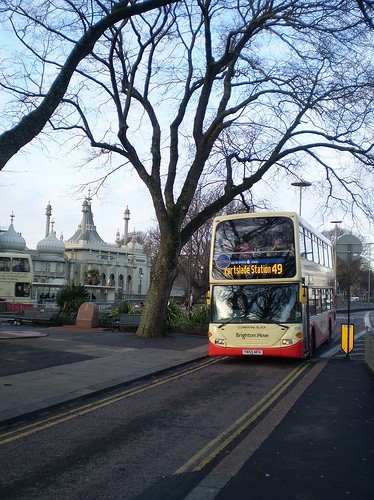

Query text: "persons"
[15, 285, 25, 296]
[39, 292, 56, 302]
[88, 292, 96, 302]
[230, 237, 295, 260]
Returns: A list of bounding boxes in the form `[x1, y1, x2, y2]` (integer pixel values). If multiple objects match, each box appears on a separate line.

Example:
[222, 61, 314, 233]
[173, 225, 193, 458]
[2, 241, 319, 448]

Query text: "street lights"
[330, 220, 342, 242]
[290, 174, 311, 215]
[366, 241, 373, 303]
[138, 270, 143, 299]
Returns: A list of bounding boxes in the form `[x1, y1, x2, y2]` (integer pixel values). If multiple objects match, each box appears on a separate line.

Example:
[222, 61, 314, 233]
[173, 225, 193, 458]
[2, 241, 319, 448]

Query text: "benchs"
[111, 313, 142, 333]
[14, 308, 61, 327]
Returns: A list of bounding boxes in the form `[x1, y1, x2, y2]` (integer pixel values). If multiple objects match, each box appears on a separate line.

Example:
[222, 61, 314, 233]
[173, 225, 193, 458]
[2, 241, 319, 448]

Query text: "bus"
[206, 211, 337, 359]
[0, 252, 38, 313]
